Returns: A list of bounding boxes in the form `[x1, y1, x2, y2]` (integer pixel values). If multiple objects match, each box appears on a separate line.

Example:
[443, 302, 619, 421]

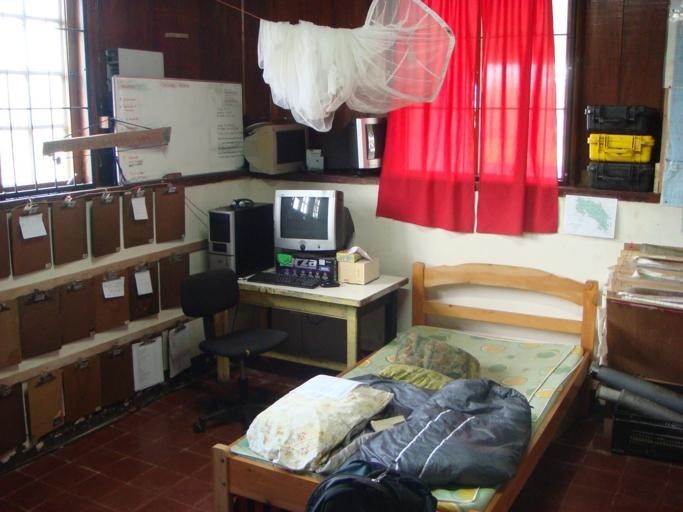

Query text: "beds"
[214, 262, 598, 512]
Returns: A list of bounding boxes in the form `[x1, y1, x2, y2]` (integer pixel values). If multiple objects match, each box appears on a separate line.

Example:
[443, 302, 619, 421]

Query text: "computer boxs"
[276, 256, 338, 281]
[209, 201, 274, 278]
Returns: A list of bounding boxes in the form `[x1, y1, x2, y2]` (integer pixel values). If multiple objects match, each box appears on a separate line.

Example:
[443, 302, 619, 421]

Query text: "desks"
[215, 269, 409, 381]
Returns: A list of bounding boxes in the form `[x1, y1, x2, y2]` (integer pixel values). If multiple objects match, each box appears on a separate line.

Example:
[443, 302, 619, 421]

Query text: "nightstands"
[606, 298, 683, 388]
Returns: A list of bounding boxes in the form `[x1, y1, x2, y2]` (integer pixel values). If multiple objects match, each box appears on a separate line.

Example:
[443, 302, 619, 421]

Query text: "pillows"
[247, 375, 394, 473]
[396, 331, 480, 381]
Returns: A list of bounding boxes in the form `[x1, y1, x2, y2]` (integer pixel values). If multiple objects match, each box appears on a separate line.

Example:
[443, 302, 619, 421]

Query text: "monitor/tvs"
[274, 190, 355, 259]
[326, 117, 387, 175]
[244, 123, 309, 175]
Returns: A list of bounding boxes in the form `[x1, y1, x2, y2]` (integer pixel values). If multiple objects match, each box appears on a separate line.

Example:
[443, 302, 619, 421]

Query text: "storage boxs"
[587, 133, 655, 164]
[584, 105, 657, 135]
[587, 162, 655, 192]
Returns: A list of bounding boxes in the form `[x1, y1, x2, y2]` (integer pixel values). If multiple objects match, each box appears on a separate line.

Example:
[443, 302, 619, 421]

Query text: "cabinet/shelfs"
[0, 184, 189, 458]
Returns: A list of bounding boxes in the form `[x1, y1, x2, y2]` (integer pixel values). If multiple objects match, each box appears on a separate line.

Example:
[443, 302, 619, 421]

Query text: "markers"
[163, 173, 181, 178]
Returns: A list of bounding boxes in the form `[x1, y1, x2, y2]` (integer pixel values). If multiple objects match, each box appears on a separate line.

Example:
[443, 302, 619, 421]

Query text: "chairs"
[181, 268, 289, 433]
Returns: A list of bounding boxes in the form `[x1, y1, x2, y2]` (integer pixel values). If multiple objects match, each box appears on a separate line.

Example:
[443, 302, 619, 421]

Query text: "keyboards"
[247, 272, 323, 289]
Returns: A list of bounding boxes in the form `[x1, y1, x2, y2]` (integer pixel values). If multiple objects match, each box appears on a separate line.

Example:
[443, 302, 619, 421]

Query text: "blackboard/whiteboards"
[111, 75, 245, 184]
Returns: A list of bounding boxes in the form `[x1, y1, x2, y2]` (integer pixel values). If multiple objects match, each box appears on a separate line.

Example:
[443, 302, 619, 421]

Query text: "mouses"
[320, 279, 340, 287]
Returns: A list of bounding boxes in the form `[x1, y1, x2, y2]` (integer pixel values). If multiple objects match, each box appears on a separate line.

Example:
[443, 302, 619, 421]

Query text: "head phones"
[231, 198, 255, 209]
